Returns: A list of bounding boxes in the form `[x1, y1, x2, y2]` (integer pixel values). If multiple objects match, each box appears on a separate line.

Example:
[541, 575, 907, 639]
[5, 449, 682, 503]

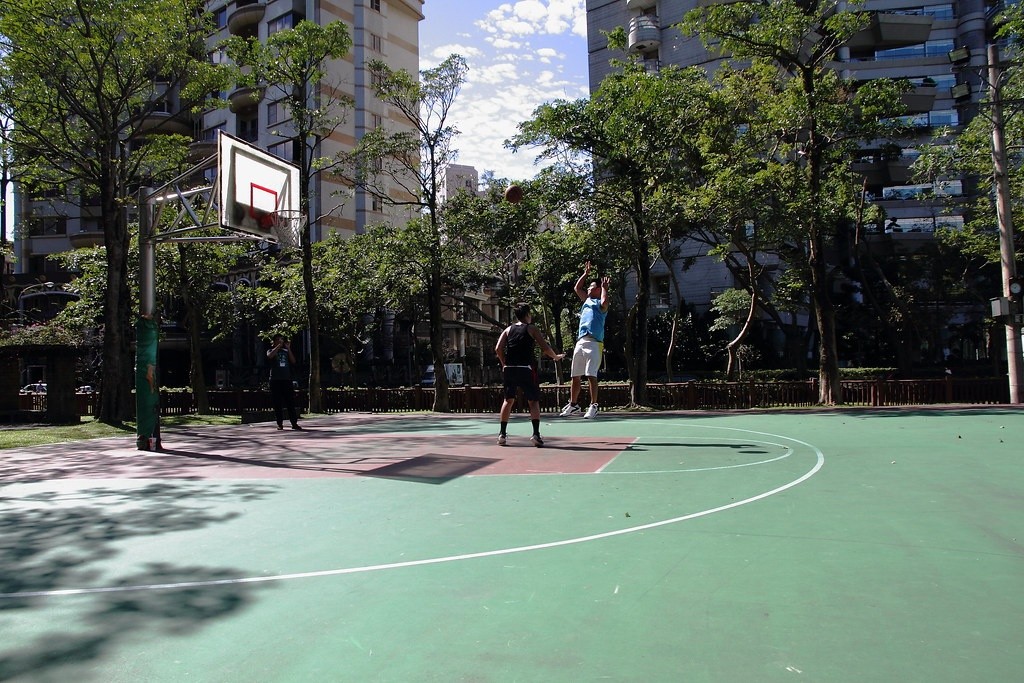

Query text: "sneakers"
[560, 404, 580, 417]
[584, 403, 598, 418]
[530, 435, 544, 447]
[497, 434, 507, 446]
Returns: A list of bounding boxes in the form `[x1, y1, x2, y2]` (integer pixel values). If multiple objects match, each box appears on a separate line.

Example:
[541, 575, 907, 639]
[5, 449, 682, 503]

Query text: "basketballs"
[506, 185, 523, 203]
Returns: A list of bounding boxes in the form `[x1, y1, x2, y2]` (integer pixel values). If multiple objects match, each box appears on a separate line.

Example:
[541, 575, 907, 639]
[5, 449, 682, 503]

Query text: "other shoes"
[292, 424, 301, 430]
[277, 425, 283, 430]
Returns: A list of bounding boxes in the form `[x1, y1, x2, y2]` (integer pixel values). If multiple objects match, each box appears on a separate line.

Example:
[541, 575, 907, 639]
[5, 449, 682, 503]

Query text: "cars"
[19, 384, 47, 408]
[262, 378, 298, 392]
[75, 385, 100, 398]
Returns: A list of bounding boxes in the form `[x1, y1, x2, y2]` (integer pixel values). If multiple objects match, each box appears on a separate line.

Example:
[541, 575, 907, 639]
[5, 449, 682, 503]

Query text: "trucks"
[421, 363, 463, 388]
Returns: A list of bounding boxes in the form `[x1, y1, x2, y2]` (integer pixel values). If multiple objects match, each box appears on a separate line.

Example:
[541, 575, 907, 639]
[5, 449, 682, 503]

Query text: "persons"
[267, 333, 302, 430]
[560, 261, 611, 419]
[495, 302, 565, 446]
